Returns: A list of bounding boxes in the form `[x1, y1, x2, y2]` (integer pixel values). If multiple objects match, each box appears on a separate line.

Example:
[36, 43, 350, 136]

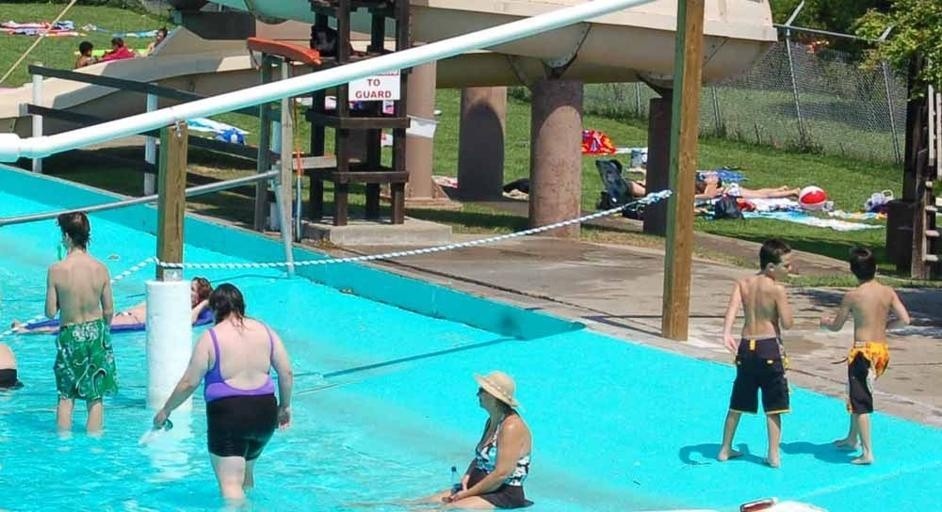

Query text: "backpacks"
[713, 196, 745, 220]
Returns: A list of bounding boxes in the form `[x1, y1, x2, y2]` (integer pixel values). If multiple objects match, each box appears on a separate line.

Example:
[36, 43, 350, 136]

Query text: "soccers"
[800, 185, 827, 210]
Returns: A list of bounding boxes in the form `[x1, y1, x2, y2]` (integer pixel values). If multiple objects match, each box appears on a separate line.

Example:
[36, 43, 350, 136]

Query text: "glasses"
[478, 387, 487, 395]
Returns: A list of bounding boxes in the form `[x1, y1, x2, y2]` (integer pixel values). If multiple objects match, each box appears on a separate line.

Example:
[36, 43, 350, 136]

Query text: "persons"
[819, 245, 911, 465]
[145, 28, 167, 57]
[718, 238, 794, 468]
[153, 283, 292, 505]
[609, 156, 801, 216]
[0, 343, 20, 390]
[44, 212, 119, 434]
[75, 41, 102, 69]
[102, 37, 135, 62]
[12, 276, 213, 337]
[343, 370, 534, 512]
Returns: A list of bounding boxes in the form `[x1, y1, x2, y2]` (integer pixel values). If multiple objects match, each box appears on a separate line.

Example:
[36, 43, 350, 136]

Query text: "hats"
[474, 371, 518, 408]
